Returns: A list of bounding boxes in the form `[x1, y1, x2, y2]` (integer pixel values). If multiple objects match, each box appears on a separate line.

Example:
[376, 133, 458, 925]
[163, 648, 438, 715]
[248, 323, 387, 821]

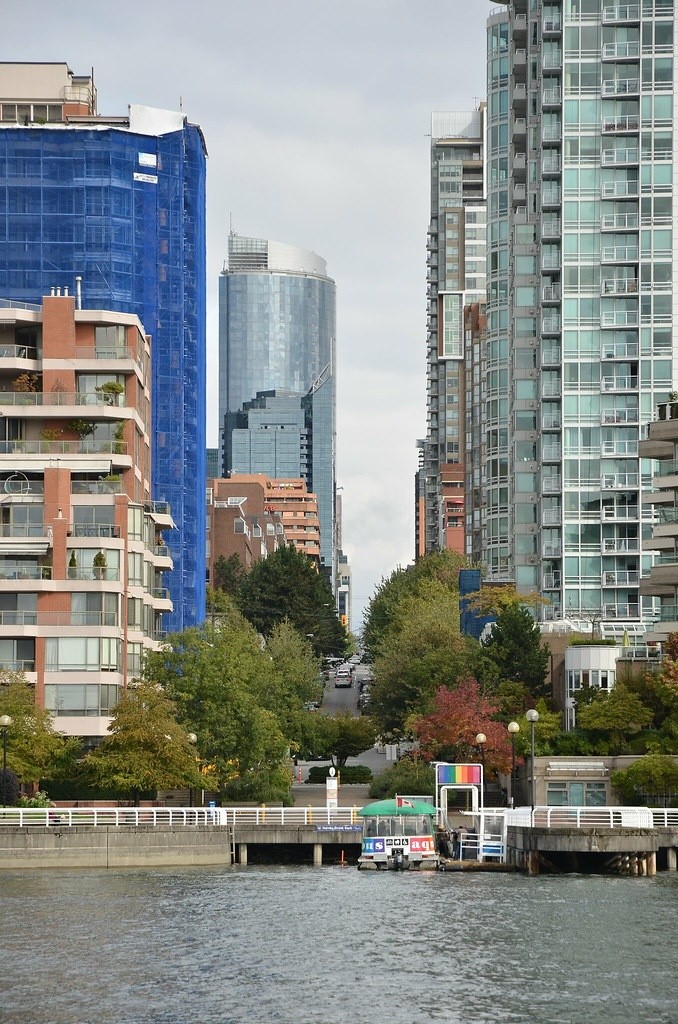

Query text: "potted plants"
[93, 552, 107, 579]
[12, 438, 24, 454]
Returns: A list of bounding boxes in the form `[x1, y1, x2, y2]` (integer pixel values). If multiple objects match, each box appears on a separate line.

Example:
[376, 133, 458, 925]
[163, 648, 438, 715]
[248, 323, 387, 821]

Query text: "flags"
[398, 798, 414, 808]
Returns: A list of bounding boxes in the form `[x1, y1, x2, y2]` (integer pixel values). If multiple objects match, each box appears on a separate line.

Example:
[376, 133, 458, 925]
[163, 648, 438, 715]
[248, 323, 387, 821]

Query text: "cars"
[298, 644, 377, 716]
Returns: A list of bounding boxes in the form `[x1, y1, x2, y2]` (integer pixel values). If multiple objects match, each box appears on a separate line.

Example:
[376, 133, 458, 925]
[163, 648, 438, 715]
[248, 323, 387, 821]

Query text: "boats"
[356, 792, 438, 873]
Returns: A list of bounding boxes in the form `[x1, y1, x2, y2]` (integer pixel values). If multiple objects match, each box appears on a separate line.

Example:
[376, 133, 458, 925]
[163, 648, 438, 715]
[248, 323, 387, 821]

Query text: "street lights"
[506, 721, 520, 810]
[186, 732, 198, 809]
[0, 714, 13, 810]
[475, 733, 491, 809]
[525, 709, 539, 808]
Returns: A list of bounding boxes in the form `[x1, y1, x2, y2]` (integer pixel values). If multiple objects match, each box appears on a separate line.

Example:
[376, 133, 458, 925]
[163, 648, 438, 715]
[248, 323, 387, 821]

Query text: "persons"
[379, 821, 386, 836]
[368, 820, 376, 836]
[49, 805, 56, 826]
[446, 826, 467, 860]
[387, 819, 396, 836]
